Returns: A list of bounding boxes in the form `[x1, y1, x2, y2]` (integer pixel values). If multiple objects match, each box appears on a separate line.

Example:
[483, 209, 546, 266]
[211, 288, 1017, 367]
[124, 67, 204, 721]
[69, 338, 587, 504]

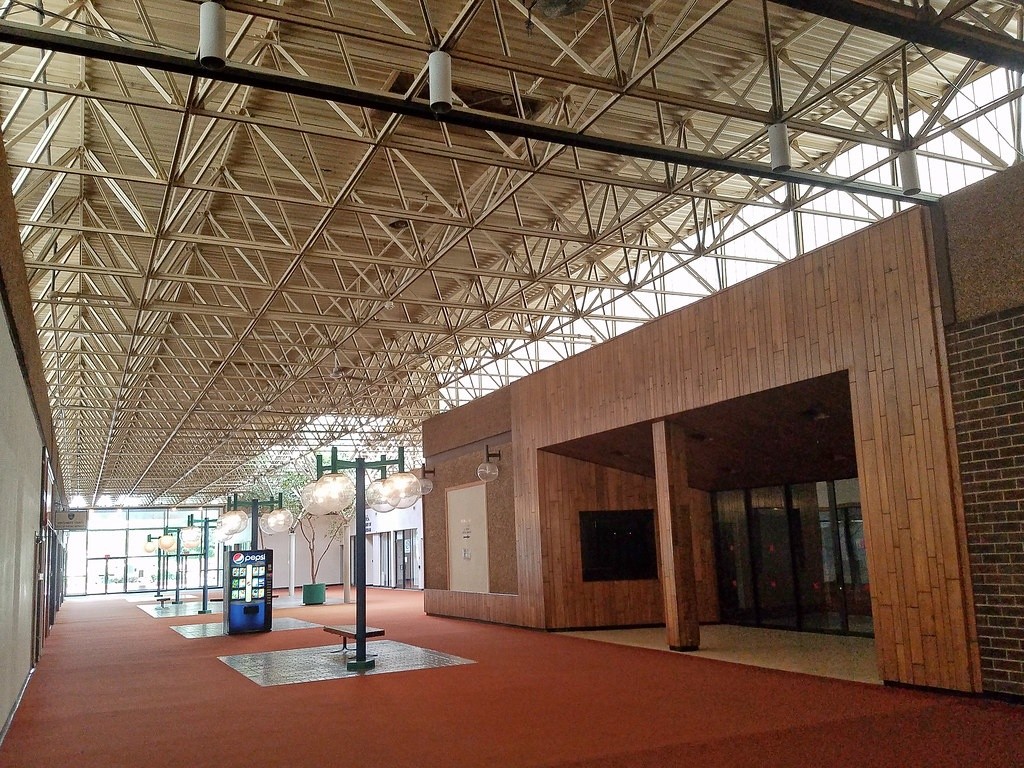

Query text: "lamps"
[476, 445, 501, 482]
[418, 463, 436, 494]
[140, 447, 423, 552]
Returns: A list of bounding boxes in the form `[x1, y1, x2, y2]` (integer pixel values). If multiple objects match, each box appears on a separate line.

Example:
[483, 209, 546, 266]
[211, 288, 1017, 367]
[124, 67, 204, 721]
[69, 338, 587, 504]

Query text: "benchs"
[323, 625, 385, 660]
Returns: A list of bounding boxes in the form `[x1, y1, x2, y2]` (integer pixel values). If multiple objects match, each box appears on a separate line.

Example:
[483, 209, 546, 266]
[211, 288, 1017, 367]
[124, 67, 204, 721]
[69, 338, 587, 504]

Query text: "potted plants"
[234, 451, 358, 604]
